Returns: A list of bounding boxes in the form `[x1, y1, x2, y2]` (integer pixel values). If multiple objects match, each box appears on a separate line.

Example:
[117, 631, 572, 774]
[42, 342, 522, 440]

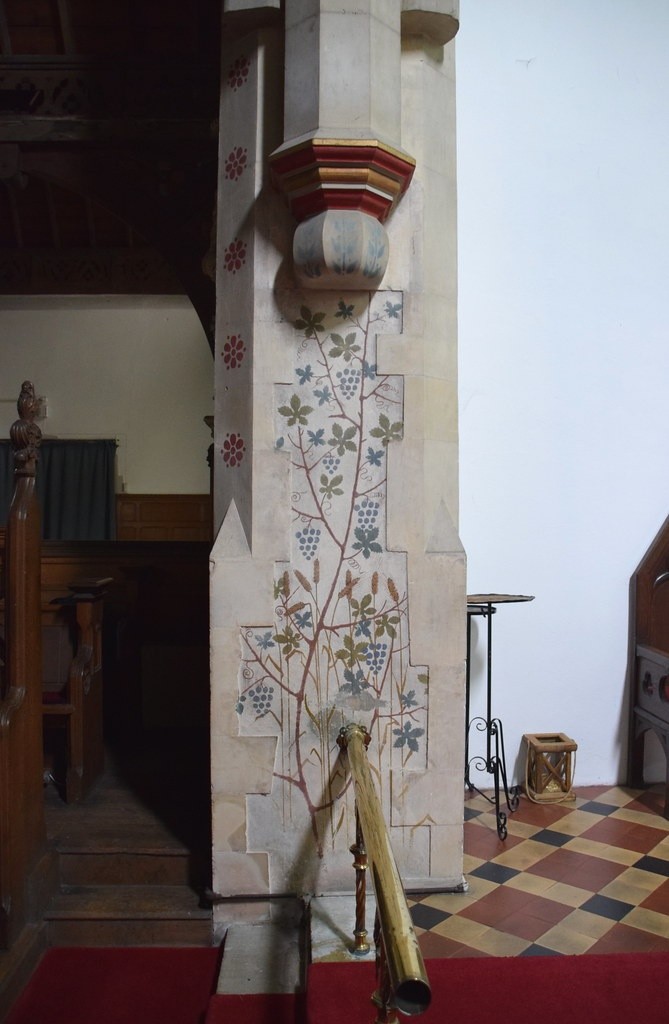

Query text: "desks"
[463, 594, 535, 841]
